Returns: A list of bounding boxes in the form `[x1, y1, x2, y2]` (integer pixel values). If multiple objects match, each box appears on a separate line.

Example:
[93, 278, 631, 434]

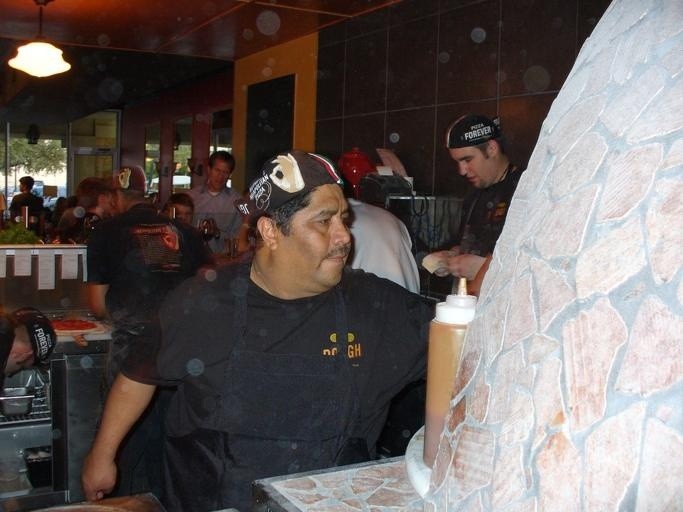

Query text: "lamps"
[3, 0, 72, 80]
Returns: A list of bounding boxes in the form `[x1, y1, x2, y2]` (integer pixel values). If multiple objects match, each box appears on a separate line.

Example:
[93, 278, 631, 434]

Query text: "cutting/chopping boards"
[49, 319, 107, 347]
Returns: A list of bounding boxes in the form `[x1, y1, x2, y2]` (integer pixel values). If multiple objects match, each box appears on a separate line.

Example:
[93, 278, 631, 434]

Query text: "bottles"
[424, 277, 479, 469]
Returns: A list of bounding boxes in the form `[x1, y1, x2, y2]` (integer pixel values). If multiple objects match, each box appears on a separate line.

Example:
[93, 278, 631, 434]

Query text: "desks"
[248, 454, 425, 512]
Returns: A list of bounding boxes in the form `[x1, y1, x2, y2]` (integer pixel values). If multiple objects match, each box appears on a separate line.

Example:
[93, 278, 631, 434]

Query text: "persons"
[80, 150, 434, 511]
[1, 302, 57, 398]
[431, 111, 526, 298]
[338, 178, 421, 298]
[2, 170, 196, 257]
[87, 164, 211, 511]
[186, 151, 255, 258]
